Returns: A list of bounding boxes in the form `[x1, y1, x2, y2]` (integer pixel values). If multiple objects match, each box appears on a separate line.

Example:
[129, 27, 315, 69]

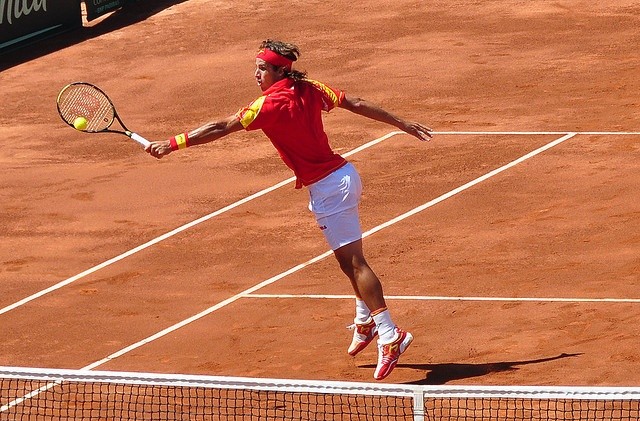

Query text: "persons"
[144, 40, 433, 380]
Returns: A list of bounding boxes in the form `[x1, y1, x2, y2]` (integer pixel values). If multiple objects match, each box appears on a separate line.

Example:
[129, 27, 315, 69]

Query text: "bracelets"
[170, 133, 189, 151]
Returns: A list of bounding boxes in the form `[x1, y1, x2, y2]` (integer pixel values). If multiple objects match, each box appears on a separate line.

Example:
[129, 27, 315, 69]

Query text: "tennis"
[72, 116, 88, 130]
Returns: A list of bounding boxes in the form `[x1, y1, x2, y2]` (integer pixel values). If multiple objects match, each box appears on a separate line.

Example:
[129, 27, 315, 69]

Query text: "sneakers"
[347, 315, 377, 356]
[373, 328, 414, 380]
